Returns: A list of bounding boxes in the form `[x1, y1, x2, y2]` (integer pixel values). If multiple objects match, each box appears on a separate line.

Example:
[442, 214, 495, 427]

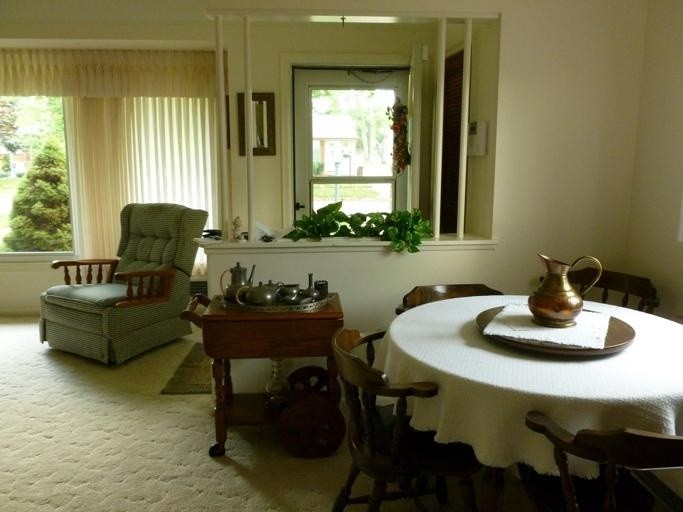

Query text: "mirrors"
[236, 91, 277, 156]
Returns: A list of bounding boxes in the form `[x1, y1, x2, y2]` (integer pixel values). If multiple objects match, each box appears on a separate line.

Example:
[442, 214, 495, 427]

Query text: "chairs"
[331, 327, 486, 512]
[517, 410, 683, 512]
[394, 283, 504, 312]
[537, 267, 658, 315]
[37, 202, 208, 368]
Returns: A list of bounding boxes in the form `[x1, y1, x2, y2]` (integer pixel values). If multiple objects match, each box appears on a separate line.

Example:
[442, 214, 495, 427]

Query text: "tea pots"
[529, 253, 603, 327]
[217, 262, 283, 307]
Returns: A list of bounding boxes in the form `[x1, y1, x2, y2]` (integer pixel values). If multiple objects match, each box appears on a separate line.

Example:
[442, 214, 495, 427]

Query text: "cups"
[281, 271, 328, 305]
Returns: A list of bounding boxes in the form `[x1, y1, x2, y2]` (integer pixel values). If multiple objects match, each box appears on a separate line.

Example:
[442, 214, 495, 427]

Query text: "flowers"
[385, 98, 410, 175]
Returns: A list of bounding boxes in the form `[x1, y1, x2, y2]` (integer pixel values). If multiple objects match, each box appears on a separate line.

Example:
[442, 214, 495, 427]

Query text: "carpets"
[159, 342, 214, 397]
